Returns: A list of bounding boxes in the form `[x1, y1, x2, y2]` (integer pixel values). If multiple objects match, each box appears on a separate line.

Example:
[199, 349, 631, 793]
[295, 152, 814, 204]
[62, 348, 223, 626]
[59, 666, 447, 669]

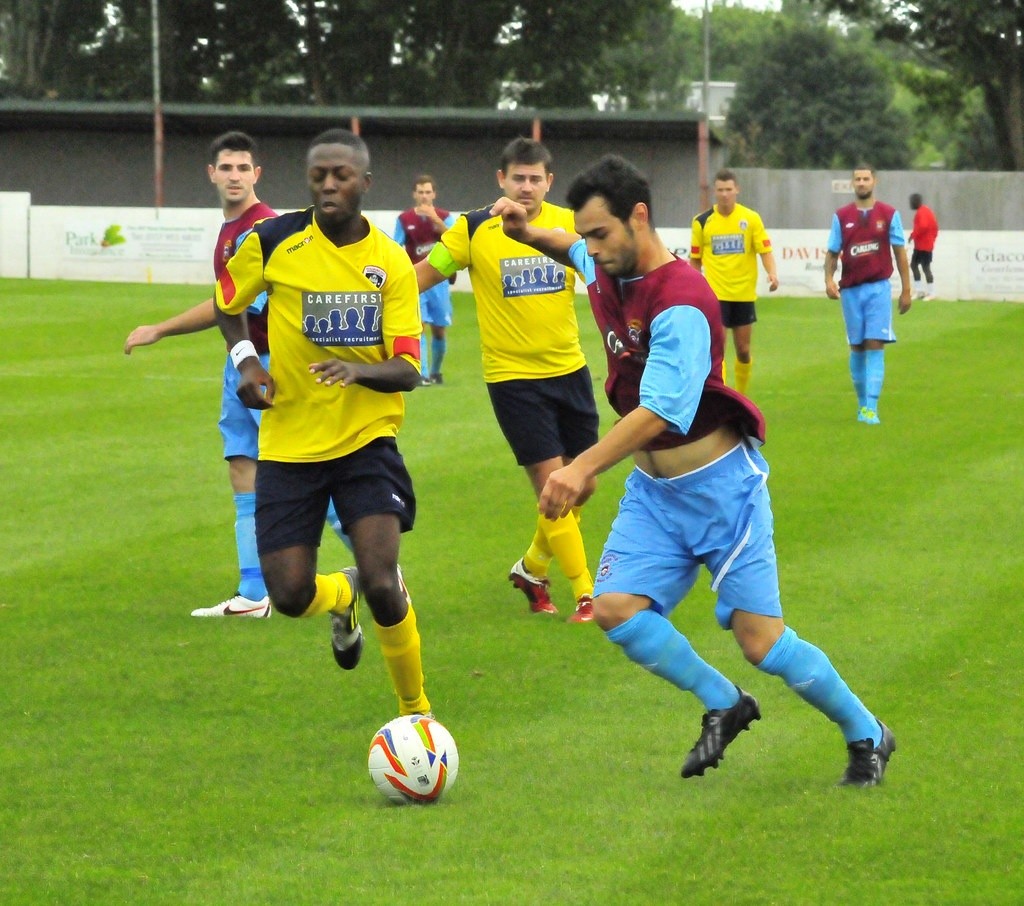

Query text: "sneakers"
[841, 717, 895, 790]
[190, 593, 272, 620]
[327, 566, 363, 670]
[571, 594, 593, 622]
[509, 556, 557, 614]
[430, 373, 442, 384]
[858, 407, 866, 421]
[417, 376, 430, 387]
[866, 409, 879, 424]
[397, 564, 411, 604]
[681, 685, 761, 779]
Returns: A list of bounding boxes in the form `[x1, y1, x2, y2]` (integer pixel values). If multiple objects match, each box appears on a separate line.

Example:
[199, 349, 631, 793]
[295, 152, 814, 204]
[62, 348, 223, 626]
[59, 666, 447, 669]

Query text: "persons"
[489, 156, 896, 789]
[414, 138, 601, 621]
[687, 171, 779, 393]
[394, 174, 457, 386]
[120, 133, 411, 616]
[908, 193, 940, 301]
[824, 164, 911, 423]
[214, 127, 432, 716]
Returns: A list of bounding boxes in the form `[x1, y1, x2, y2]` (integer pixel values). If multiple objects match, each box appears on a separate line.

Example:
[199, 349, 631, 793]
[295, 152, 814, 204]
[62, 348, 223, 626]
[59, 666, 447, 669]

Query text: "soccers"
[368, 713, 460, 806]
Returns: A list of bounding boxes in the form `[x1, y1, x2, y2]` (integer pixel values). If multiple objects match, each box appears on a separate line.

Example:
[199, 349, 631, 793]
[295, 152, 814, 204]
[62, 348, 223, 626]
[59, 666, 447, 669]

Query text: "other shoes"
[923, 293, 935, 302]
[912, 290, 920, 300]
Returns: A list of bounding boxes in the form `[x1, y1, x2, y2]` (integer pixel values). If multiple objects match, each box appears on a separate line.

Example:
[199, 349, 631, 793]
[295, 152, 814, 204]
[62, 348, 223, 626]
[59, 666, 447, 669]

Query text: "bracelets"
[230, 340, 260, 370]
